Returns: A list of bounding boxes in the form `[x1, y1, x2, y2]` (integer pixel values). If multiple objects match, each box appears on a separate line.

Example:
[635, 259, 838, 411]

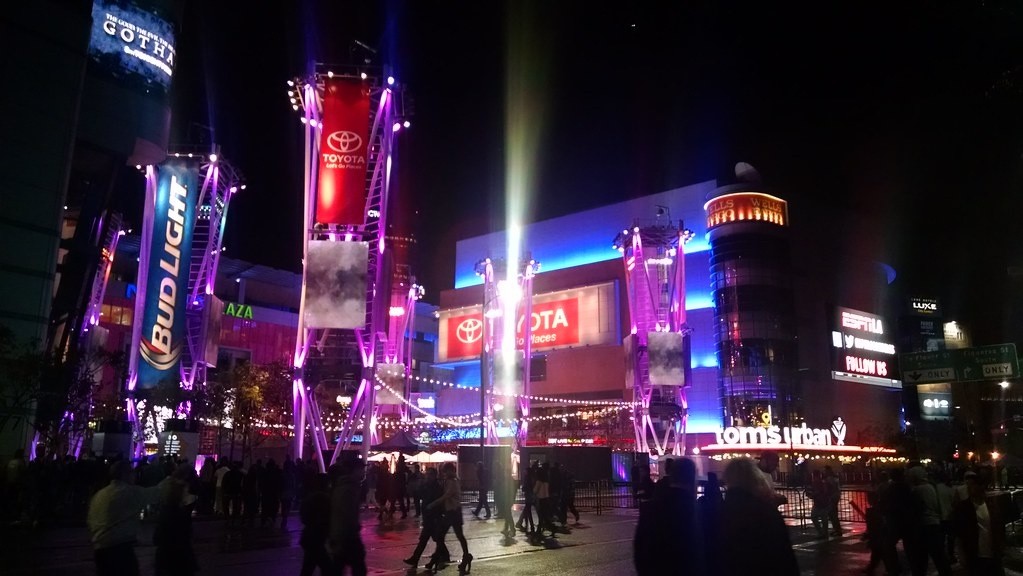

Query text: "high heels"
[425, 554, 442, 571]
[458, 554, 473, 572]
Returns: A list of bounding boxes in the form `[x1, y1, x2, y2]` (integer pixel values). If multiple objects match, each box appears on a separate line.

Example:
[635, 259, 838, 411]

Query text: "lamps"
[286, 78, 304, 110]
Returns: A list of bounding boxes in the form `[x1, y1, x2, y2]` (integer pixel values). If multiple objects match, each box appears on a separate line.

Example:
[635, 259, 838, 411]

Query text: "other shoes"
[501, 520, 568, 537]
[484, 515, 491, 517]
[438, 551, 449, 562]
[575, 511, 579, 521]
[403, 556, 419, 565]
[472, 510, 478, 516]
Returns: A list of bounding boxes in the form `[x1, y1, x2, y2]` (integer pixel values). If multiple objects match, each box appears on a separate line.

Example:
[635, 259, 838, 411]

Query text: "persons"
[516, 463, 579, 537]
[806, 465, 842, 538]
[861, 461, 1023, 576]
[471, 460, 515, 535]
[197, 455, 369, 576]
[634, 451, 801, 576]
[0, 444, 198, 576]
[364, 456, 472, 570]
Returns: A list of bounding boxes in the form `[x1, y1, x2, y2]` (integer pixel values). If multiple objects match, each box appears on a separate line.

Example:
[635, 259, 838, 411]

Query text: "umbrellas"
[369, 430, 430, 455]
